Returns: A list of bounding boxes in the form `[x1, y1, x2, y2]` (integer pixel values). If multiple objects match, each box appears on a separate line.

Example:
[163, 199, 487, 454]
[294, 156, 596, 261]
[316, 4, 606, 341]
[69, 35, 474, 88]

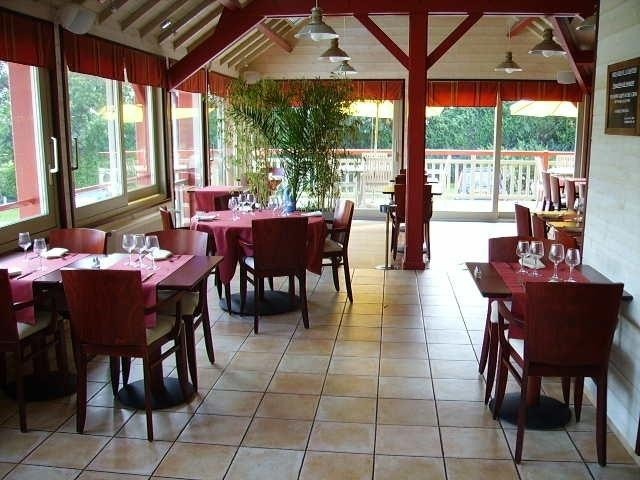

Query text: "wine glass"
[529, 241, 544, 278]
[548, 243, 564, 282]
[228, 199, 236, 221]
[122, 234, 136, 268]
[565, 249, 580, 281]
[145, 235, 159, 272]
[268, 198, 278, 219]
[247, 194, 255, 213]
[33, 238, 47, 273]
[231, 197, 240, 219]
[238, 194, 246, 212]
[18, 232, 32, 262]
[134, 234, 145, 269]
[515, 241, 528, 274]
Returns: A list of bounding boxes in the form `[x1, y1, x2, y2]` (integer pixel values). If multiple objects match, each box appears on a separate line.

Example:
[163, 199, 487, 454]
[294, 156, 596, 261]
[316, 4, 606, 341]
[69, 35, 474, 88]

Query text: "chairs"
[331, 15, 359, 76]
[318, 40, 353, 63]
[396, 183, 432, 262]
[397, 175, 428, 185]
[493, 18, 528, 74]
[552, 177, 560, 211]
[46, 229, 112, 255]
[531, 214, 549, 237]
[292, 2, 339, 42]
[158, 204, 233, 315]
[563, 182, 574, 213]
[513, 203, 529, 240]
[61, 270, 193, 442]
[399, 167, 428, 174]
[492, 283, 627, 467]
[145, 229, 216, 366]
[240, 216, 310, 333]
[290, 198, 356, 304]
[539, 171, 550, 208]
[1, 269, 62, 434]
[554, 228, 574, 250]
[493, 236, 558, 266]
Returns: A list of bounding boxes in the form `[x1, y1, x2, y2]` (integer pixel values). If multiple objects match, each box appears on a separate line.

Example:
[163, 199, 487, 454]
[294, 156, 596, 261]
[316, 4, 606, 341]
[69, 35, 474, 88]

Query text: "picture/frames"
[605, 59, 640, 133]
